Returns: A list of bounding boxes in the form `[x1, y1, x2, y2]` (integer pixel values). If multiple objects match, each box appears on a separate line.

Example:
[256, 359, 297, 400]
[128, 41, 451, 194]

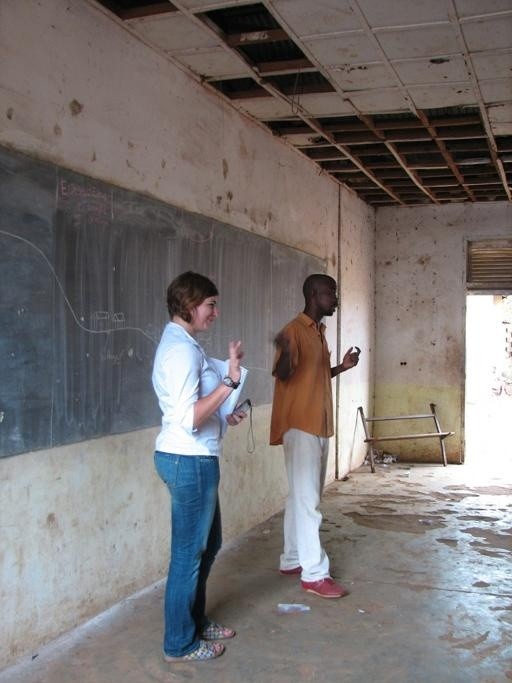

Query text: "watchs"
[221, 375, 241, 391]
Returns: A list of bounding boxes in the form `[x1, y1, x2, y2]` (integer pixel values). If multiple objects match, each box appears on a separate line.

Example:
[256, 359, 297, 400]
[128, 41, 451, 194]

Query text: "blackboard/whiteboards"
[0, 143, 327, 459]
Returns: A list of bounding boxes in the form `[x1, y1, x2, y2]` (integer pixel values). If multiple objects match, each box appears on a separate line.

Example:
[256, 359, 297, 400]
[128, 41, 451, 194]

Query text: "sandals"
[163, 640, 225, 661]
[200, 623, 234, 640]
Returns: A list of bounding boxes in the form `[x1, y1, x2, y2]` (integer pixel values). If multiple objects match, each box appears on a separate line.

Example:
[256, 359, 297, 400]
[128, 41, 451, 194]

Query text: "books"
[207, 354, 249, 440]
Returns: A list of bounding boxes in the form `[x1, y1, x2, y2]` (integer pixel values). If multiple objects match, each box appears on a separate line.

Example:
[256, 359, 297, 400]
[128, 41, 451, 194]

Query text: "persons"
[266, 272, 361, 599]
[151, 268, 254, 664]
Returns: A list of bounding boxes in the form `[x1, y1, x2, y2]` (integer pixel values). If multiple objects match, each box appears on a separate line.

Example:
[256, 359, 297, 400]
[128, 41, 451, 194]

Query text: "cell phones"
[235, 398, 251, 417]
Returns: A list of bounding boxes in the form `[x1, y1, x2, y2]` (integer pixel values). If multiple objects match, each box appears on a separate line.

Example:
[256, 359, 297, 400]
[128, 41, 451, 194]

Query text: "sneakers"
[279, 558, 347, 599]
